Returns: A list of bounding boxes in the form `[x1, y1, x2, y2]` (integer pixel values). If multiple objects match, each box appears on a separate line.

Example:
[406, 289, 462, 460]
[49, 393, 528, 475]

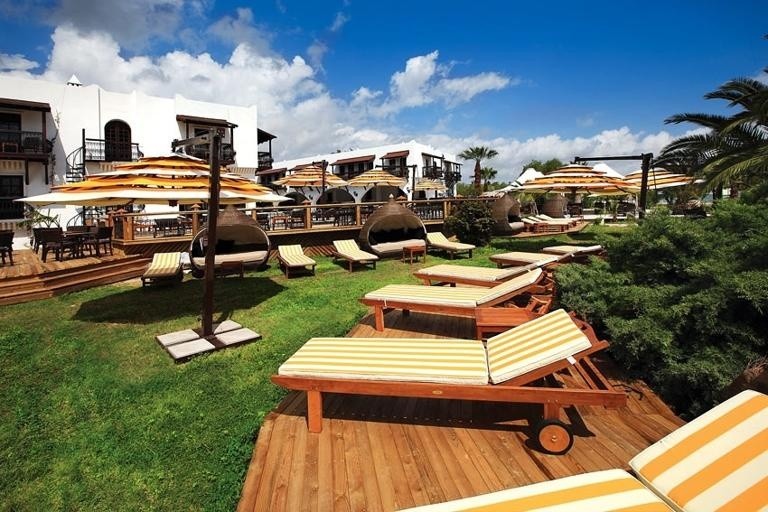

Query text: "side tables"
[219, 260, 243, 283]
[473, 305, 530, 339]
[402, 246, 426, 265]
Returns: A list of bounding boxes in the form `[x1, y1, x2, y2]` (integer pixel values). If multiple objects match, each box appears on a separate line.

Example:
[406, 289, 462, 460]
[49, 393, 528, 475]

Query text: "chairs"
[521, 212, 584, 232]
[314, 205, 356, 226]
[141, 251, 183, 289]
[253, 211, 312, 231]
[413, 256, 556, 288]
[426, 231, 475, 260]
[277, 244, 315, 278]
[489, 245, 601, 270]
[0, 225, 115, 267]
[330, 238, 379, 273]
[150, 217, 200, 236]
[384, 389, 768, 511]
[541, 244, 608, 256]
[270, 308, 627, 455]
[356, 267, 546, 332]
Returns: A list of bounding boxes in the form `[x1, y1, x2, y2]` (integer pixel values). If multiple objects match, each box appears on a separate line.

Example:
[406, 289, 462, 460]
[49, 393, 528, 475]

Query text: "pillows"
[215, 239, 234, 254]
[405, 227, 417, 239]
[390, 226, 405, 241]
[368, 230, 381, 245]
[414, 226, 424, 240]
[378, 228, 390, 244]
[193, 242, 207, 257]
